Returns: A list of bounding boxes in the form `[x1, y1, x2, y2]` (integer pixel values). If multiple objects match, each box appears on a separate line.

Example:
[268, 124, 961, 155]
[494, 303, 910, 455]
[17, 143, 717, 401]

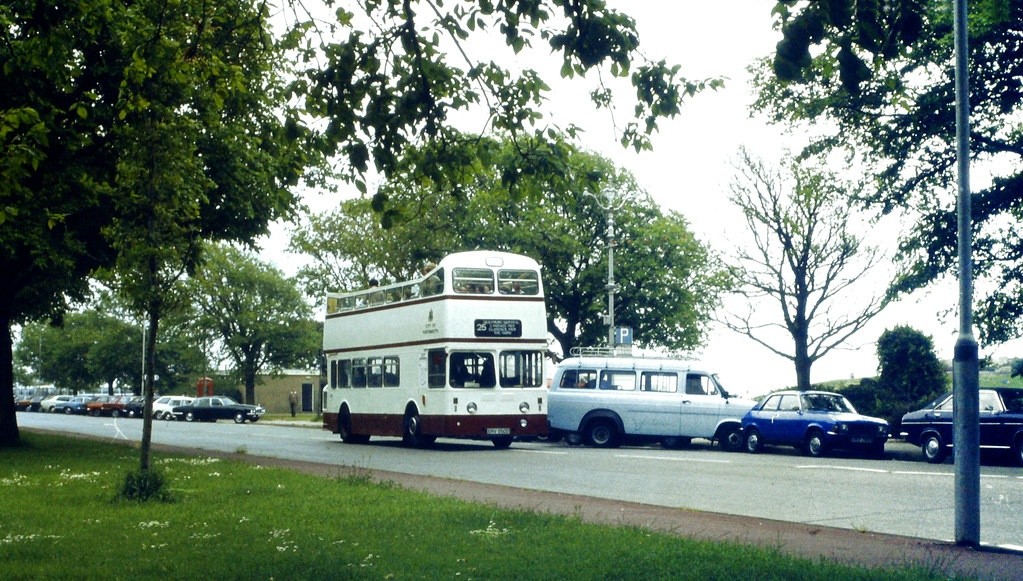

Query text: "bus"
[321, 249, 548, 448]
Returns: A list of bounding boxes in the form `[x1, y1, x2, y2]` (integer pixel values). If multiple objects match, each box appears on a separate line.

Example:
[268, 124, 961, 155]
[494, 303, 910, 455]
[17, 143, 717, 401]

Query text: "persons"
[289, 385, 298, 417]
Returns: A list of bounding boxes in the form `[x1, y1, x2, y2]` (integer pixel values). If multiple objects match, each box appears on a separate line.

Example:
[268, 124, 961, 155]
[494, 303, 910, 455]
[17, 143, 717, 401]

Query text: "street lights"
[580, 181, 638, 344]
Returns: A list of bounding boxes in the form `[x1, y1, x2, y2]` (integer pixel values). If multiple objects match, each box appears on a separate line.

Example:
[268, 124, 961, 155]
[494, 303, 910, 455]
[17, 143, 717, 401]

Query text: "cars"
[899, 387, 1023, 468]
[13, 391, 194, 421]
[741, 388, 889, 460]
[171, 395, 266, 424]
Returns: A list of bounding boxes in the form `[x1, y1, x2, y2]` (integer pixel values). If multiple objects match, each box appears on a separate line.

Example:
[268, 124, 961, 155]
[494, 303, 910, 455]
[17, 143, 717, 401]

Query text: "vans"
[548, 346, 761, 452]
